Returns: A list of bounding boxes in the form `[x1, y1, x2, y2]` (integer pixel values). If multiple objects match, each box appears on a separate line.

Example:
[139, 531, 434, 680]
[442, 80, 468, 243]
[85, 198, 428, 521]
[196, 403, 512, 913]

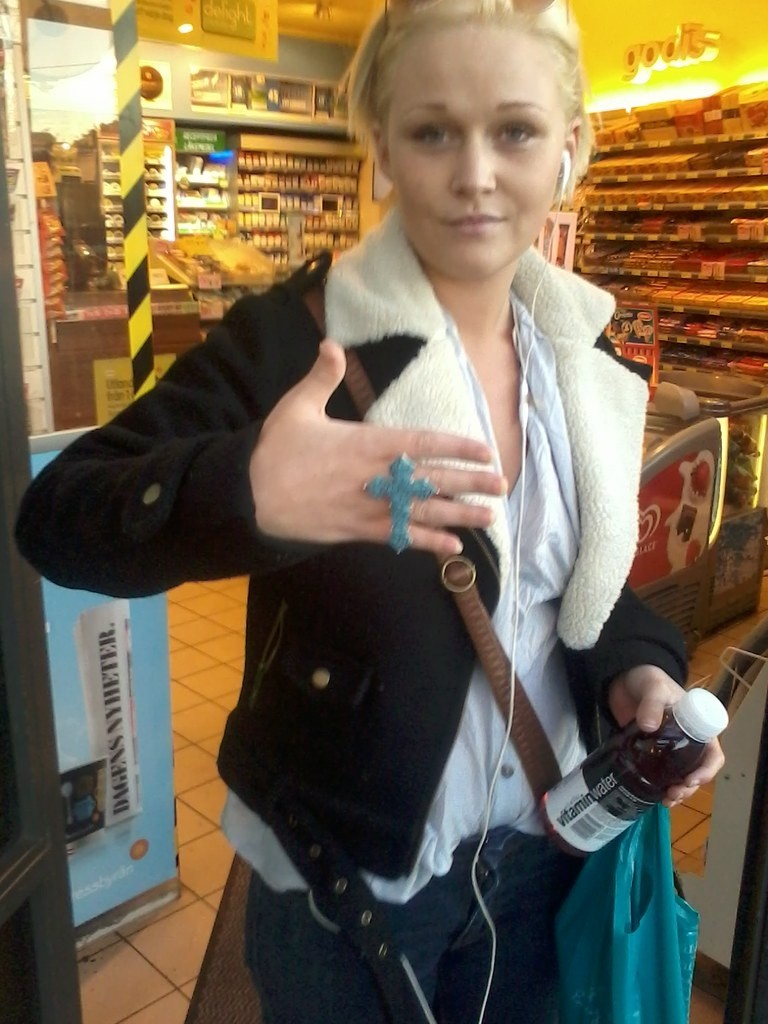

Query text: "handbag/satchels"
[555, 799, 701, 1024]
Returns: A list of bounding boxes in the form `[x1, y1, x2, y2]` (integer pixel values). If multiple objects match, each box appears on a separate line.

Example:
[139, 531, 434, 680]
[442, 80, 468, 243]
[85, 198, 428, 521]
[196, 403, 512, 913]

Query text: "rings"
[360, 450, 441, 557]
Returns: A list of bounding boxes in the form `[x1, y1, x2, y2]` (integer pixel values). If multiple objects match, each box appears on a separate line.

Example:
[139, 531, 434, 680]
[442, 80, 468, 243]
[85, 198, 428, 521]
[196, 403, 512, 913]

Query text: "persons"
[16, 0, 725, 1024]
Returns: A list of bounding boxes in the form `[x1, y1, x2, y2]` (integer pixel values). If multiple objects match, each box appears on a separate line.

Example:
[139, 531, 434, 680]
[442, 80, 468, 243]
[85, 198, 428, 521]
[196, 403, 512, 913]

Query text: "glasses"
[385, 0, 569, 27]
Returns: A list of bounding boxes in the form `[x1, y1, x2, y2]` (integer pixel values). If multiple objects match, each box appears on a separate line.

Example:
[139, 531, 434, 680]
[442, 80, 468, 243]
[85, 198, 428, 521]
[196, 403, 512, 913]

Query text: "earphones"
[556, 149, 571, 202]
